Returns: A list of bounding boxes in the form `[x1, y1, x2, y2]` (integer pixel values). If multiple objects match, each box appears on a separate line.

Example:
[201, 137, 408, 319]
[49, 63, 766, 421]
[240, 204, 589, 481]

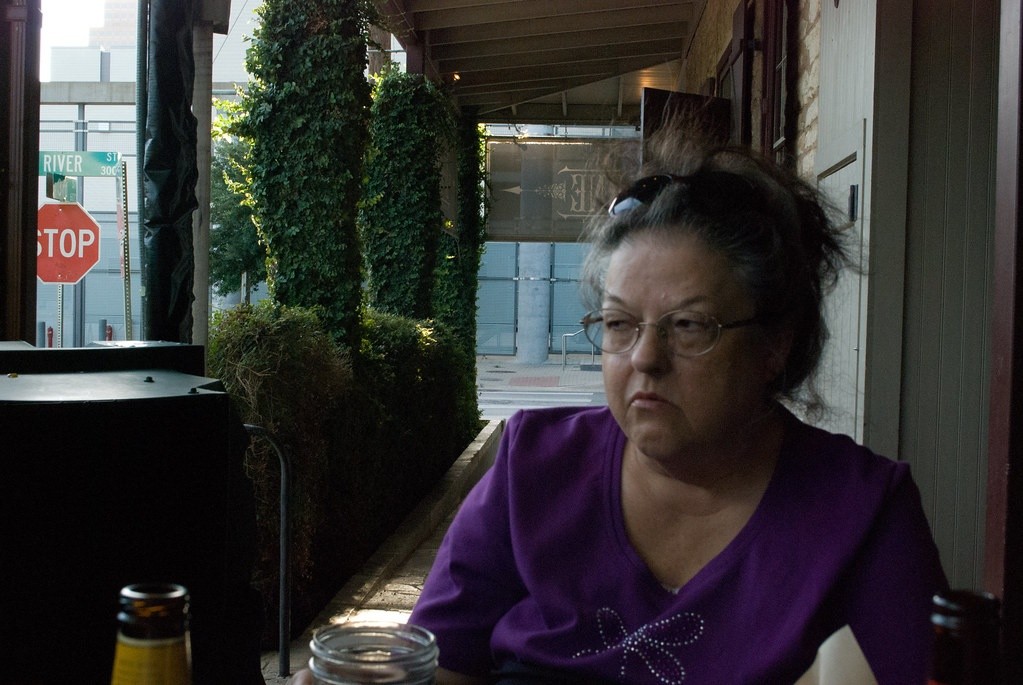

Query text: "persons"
[288, 120, 950, 685]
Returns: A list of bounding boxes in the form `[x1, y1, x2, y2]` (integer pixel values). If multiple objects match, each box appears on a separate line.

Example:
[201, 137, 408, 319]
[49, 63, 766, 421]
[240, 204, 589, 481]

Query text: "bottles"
[925, 589, 1007, 684]
[111, 583, 193, 685]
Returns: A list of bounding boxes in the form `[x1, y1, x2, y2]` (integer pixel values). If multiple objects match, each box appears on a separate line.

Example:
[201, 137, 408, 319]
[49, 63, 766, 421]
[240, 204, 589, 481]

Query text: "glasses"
[579, 308, 759, 358]
[608, 169, 758, 218]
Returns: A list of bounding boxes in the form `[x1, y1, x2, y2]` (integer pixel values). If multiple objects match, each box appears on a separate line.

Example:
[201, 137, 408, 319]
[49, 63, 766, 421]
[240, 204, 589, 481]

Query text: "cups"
[308, 621, 439, 685]
